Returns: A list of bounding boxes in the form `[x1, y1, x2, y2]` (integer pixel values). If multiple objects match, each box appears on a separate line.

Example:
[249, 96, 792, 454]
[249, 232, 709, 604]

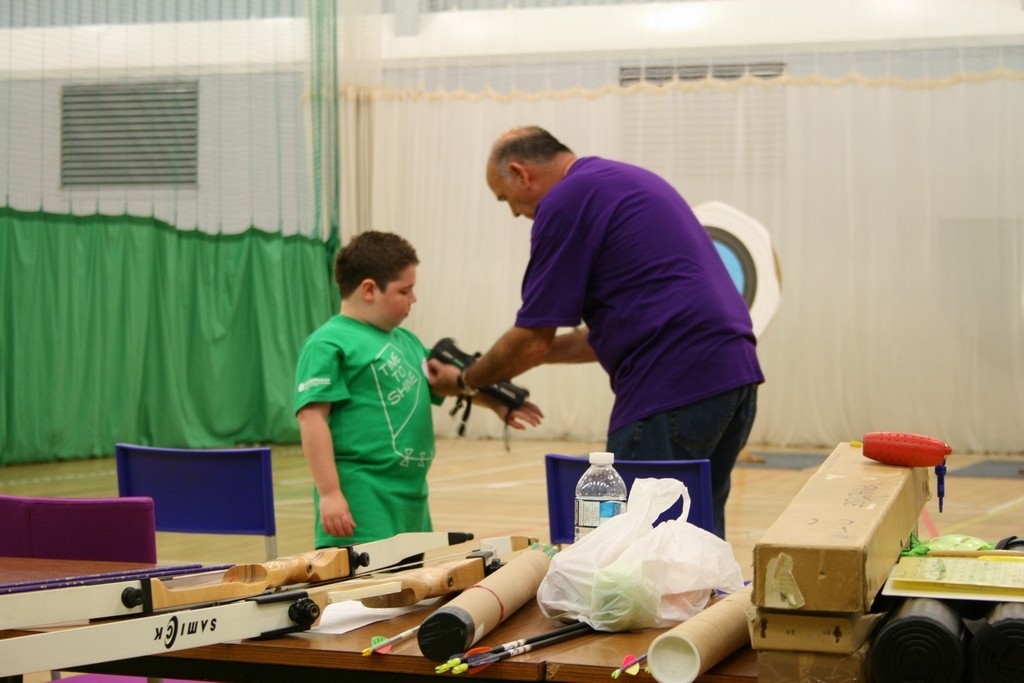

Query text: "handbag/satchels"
[536, 476, 745, 633]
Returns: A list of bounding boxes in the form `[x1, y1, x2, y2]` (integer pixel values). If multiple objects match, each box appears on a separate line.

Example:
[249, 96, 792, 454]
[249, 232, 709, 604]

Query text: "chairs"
[113, 443, 277, 562]
[0, 493, 157, 583]
[543, 452, 714, 553]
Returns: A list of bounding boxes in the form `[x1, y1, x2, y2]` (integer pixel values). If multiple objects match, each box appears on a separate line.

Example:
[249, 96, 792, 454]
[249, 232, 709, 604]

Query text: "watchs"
[457, 372, 480, 396]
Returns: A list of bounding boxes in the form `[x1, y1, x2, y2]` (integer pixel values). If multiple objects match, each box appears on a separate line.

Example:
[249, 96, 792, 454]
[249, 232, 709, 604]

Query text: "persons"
[293, 230, 546, 553]
[427, 125, 767, 541]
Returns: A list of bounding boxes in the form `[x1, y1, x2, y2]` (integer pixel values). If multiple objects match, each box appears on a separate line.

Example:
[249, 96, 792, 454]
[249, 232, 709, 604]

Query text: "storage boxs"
[748, 441, 934, 683]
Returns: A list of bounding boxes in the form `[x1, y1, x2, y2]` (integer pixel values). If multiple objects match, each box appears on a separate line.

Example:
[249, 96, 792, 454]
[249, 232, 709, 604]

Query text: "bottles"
[573, 452, 628, 543]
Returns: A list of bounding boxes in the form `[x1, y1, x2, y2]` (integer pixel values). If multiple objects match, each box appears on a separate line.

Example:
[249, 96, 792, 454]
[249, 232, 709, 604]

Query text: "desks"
[547, 624, 863, 683]
[0, 596, 612, 683]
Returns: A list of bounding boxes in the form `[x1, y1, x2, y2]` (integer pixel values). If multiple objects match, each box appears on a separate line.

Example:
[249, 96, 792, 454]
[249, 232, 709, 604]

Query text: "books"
[893, 556, 1024, 596]
